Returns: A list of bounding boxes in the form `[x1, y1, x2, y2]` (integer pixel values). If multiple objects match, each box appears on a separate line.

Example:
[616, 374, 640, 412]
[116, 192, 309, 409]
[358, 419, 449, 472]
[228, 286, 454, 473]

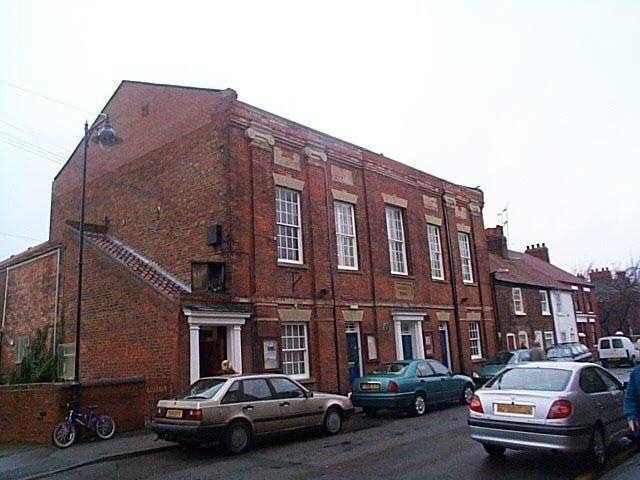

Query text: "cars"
[153, 374, 354, 453]
[479, 341, 594, 386]
[469, 361, 640, 472]
[356, 359, 475, 416]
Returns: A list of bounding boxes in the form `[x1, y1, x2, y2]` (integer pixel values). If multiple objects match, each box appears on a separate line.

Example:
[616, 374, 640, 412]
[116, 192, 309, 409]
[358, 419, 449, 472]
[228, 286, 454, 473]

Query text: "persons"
[521, 342, 528, 349]
[622, 339, 640, 433]
[218, 358, 241, 378]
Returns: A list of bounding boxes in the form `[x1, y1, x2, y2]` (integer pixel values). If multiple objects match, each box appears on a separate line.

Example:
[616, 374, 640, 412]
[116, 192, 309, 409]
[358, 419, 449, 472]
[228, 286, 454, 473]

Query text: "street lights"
[71, 114, 123, 399]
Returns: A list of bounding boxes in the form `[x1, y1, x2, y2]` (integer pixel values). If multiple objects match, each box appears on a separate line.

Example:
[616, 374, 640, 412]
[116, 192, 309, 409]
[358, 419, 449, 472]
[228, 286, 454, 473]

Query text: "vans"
[597, 331, 640, 367]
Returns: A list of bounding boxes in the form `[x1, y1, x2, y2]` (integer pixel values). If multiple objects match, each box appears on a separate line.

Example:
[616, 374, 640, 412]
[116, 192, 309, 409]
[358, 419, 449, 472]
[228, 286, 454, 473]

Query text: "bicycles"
[52, 401, 117, 449]
